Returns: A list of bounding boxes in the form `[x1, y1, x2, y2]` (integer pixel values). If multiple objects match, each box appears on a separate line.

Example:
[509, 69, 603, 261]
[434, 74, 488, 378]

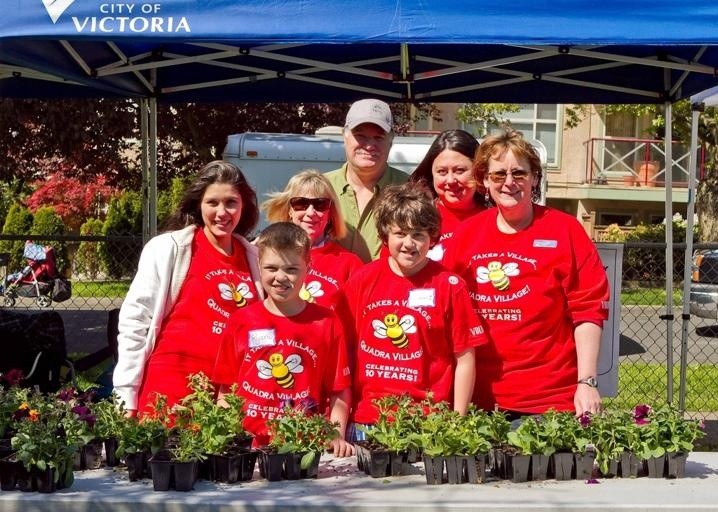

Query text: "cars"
[679, 248, 717, 320]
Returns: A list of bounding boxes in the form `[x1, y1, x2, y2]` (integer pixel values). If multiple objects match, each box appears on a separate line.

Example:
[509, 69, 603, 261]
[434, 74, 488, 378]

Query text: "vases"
[602, 452, 619, 479]
[286, 451, 322, 481]
[647, 453, 666, 478]
[82, 440, 104, 474]
[0, 439, 16, 491]
[18, 459, 71, 494]
[621, 451, 639, 480]
[255, 447, 284, 482]
[578, 451, 596, 484]
[668, 451, 687, 478]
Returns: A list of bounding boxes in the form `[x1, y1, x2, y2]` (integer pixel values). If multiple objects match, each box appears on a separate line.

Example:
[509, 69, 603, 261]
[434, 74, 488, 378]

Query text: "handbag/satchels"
[50, 278, 71, 302]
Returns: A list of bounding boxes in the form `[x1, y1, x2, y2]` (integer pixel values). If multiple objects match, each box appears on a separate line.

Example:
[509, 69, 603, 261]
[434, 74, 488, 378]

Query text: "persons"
[331, 182, 489, 443]
[112, 160, 263, 429]
[440, 128, 609, 421]
[209, 221, 356, 458]
[259, 168, 363, 308]
[407, 129, 491, 253]
[323, 99, 413, 255]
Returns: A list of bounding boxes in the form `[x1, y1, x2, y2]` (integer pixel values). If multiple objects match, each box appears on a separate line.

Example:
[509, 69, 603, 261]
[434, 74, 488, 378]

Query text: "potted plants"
[444, 410, 467, 484]
[149, 399, 199, 492]
[421, 412, 444, 485]
[490, 410, 530, 482]
[105, 391, 129, 466]
[354, 392, 420, 478]
[119, 393, 167, 482]
[464, 401, 491, 483]
[525, 415, 555, 480]
[551, 408, 575, 480]
[190, 372, 259, 484]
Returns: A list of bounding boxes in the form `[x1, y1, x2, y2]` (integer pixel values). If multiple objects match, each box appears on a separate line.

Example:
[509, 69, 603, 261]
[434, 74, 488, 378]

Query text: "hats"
[343, 98, 394, 132]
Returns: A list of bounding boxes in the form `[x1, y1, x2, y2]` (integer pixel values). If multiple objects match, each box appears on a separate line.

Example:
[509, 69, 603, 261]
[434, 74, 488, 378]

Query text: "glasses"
[287, 197, 333, 211]
[487, 169, 534, 182]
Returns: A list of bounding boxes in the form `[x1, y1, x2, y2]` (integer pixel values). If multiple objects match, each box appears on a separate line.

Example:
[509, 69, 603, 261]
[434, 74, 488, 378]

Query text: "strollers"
[3, 243, 59, 308]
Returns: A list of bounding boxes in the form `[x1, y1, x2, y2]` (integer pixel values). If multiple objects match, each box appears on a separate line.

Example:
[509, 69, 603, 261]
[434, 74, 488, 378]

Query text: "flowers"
[615, 411, 640, 452]
[56, 385, 106, 441]
[266, 398, 293, 451]
[594, 414, 618, 450]
[0, 370, 28, 439]
[663, 400, 707, 453]
[641, 403, 667, 458]
[289, 395, 340, 453]
[11, 405, 75, 473]
[572, 411, 593, 450]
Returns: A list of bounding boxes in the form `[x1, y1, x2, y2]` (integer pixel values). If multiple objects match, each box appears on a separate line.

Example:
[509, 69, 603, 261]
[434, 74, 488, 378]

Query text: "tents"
[0, 0, 717, 423]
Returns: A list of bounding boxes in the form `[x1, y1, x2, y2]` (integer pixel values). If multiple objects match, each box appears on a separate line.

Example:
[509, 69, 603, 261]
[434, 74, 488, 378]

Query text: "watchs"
[577, 375, 599, 388]
